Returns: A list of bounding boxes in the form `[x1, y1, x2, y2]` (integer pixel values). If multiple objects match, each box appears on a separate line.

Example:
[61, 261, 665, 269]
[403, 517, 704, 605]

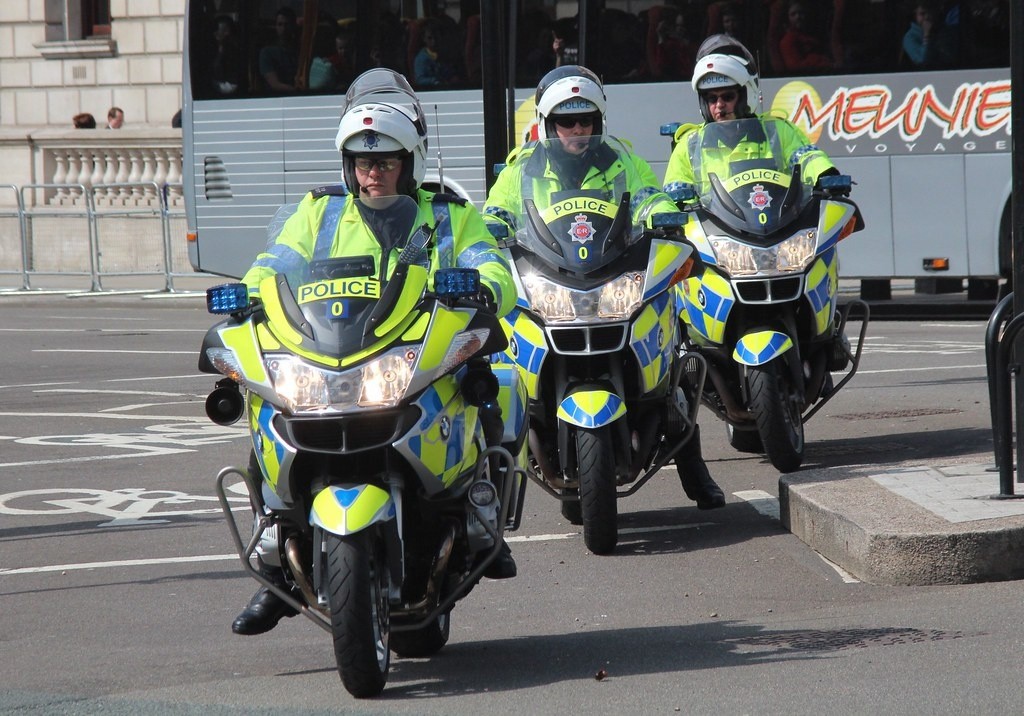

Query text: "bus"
[181, 0, 1023, 289]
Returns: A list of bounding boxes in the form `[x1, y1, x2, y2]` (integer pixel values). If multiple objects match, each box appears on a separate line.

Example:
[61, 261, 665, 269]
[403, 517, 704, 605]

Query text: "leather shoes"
[481, 544, 517, 578]
[232, 568, 308, 635]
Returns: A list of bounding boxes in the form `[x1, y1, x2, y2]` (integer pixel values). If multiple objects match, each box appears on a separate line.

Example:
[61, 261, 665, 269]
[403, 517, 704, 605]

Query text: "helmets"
[691, 46, 760, 122]
[334, 90, 428, 196]
[536, 64, 607, 151]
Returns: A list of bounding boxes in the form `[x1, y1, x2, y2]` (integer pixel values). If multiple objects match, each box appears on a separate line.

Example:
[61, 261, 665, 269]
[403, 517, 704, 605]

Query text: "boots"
[673, 424, 725, 509]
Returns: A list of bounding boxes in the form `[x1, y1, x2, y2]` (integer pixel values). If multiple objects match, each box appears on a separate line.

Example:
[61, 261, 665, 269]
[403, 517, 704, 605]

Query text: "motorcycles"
[497, 139, 708, 558]
[198, 194, 523, 699]
[670, 116, 873, 474]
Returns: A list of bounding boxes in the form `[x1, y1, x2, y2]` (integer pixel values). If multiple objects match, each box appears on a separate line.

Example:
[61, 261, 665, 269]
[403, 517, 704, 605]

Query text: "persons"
[664, 35, 852, 371]
[103, 107, 123, 129]
[72, 114, 96, 129]
[233, 68, 519, 636]
[485, 66, 726, 509]
[200, 0, 1011, 101]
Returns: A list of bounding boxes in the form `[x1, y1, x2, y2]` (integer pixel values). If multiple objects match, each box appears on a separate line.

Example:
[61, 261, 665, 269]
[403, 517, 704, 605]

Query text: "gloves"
[823, 171, 852, 198]
[228, 300, 258, 318]
[460, 287, 494, 312]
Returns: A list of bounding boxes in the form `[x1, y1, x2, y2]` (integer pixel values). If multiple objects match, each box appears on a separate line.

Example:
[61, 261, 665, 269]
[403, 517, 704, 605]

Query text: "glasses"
[353, 158, 400, 172]
[706, 91, 737, 103]
[552, 117, 593, 128]
